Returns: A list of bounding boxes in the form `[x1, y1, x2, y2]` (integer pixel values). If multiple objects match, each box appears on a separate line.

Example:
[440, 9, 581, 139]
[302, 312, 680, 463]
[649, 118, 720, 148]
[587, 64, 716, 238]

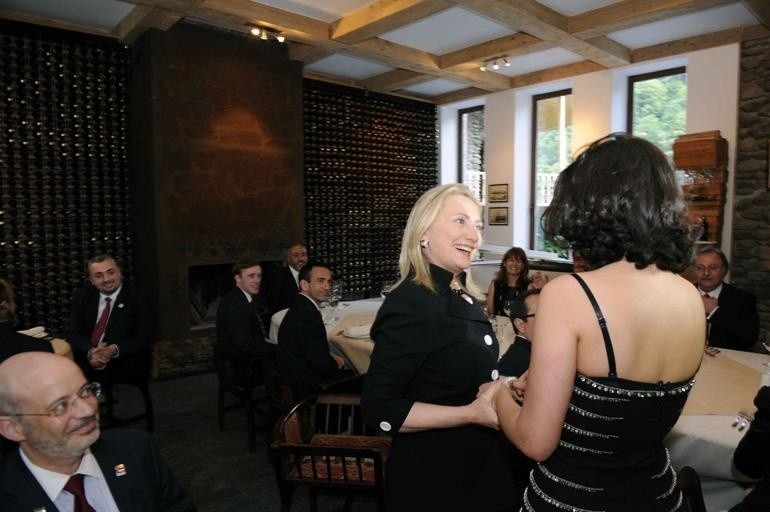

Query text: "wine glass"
[760, 331, 770, 368]
[503, 300, 515, 326]
[380, 280, 394, 299]
[329, 280, 343, 320]
[480, 304, 495, 335]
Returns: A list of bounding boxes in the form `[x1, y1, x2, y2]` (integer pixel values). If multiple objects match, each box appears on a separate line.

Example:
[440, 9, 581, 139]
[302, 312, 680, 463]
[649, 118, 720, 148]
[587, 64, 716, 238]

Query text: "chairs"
[269, 393, 392, 512]
[255, 333, 356, 425]
[98, 354, 155, 434]
[213, 342, 272, 452]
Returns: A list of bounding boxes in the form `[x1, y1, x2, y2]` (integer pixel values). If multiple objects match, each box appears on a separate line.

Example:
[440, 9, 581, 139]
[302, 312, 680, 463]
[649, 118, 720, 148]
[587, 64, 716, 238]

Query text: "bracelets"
[510, 374, 515, 383]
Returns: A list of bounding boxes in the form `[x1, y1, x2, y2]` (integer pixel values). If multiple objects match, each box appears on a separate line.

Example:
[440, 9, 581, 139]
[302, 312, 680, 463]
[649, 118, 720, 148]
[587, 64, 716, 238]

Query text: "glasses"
[2, 381, 103, 418]
[695, 261, 723, 271]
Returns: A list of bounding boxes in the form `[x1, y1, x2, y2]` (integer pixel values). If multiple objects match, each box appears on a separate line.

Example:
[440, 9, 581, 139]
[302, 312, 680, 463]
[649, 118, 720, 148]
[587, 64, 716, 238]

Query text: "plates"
[342, 332, 371, 339]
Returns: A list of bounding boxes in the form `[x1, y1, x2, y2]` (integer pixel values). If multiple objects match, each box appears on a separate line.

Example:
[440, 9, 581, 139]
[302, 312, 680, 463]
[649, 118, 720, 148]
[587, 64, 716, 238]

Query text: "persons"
[361, 183, 516, 512]
[477, 131, 708, 510]
[690, 248, 759, 351]
[278, 264, 353, 424]
[0, 352, 198, 511]
[498, 290, 539, 383]
[69, 255, 150, 384]
[727, 385, 769, 510]
[264, 245, 309, 311]
[220, 262, 273, 387]
[1, 282, 54, 511]
[532, 248, 583, 290]
[488, 246, 528, 313]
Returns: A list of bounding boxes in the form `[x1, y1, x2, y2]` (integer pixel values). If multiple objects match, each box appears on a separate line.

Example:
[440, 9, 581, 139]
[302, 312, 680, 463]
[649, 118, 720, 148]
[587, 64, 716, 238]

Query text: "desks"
[269, 298, 770, 512]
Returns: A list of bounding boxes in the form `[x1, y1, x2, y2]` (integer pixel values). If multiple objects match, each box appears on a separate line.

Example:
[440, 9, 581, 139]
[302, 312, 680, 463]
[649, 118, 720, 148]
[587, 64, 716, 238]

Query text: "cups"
[704, 322, 711, 349]
[321, 306, 334, 325]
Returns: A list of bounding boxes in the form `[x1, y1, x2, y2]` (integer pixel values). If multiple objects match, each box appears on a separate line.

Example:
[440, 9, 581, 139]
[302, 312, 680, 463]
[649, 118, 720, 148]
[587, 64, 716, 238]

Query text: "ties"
[90, 296, 111, 348]
[63, 474, 97, 512]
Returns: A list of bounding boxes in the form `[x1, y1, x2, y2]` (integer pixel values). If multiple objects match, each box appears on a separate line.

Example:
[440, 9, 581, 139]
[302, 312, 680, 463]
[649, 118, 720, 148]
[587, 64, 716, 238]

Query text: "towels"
[343, 324, 372, 337]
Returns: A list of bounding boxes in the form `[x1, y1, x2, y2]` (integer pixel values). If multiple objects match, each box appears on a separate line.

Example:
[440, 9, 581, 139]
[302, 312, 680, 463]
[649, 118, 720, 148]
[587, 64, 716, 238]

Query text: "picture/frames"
[487, 184, 508, 203]
[488, 207, 508, 225]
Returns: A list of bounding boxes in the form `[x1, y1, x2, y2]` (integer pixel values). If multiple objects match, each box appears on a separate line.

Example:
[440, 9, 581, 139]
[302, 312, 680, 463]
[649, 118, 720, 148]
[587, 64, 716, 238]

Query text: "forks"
[732, 411, 747, 427]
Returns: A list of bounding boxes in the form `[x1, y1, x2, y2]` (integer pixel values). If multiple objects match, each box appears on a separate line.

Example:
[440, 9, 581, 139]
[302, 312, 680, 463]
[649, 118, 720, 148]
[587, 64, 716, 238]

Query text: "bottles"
[699, 216, 710, 241]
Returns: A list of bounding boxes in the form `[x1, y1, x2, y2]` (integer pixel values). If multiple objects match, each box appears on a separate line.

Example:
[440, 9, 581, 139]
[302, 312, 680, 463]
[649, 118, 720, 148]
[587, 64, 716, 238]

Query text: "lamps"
[243, 22, 286, 43]
[479, 56, 510, 72]
